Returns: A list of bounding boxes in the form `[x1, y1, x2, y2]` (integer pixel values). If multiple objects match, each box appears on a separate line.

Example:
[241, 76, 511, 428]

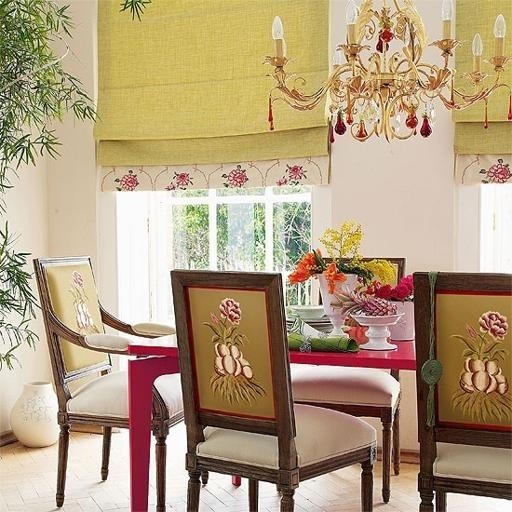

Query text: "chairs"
[289, 362, 402, 505]
[412, 271, 512, 512]
[166, 268, 377, 512]
[31, 255, 188, 512]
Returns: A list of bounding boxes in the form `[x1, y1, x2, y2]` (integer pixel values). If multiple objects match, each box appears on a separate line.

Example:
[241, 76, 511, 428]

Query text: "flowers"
[67, 270, 96, 332]
[479, 158, 512, 184]
[287, 221, 397, 294]
[222, 165, 249, 188]
[276, 164, 307, 186]
[448, 310, 512, 424]
[164, 171, 193, 191]
[201, 297, 267, 407]
[114, 170, 139, 191]
[374, 273, 414, 302]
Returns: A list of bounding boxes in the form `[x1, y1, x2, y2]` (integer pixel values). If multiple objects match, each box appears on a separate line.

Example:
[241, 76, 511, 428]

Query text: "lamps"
[262, 0, 512, 144]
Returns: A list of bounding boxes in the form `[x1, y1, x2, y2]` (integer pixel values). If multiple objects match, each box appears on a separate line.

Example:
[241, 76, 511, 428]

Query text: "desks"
[127, 326, 417, 511]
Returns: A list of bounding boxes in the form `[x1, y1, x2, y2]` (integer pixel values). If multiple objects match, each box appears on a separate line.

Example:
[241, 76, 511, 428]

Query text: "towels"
[286, 316, 323, 335]
[287, 333, 360, 353]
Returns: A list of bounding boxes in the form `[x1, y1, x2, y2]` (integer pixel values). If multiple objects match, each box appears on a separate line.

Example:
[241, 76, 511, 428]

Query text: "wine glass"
[350, 313, 406, 351]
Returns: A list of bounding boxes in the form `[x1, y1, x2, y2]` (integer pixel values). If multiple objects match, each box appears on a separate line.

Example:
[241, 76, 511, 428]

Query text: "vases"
[9, 380, 61, 447]
[387, 301, 415, 341]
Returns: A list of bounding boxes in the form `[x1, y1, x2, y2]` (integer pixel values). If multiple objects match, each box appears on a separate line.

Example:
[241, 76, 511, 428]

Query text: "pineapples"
[329, 287, 398, 316]
